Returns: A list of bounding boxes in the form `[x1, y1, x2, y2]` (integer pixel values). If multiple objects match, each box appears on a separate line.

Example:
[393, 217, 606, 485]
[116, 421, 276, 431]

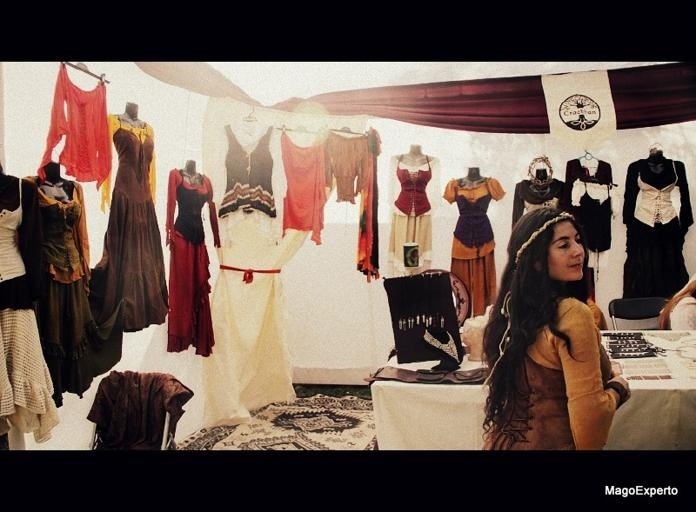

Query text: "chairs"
[86, 368, 194, 449]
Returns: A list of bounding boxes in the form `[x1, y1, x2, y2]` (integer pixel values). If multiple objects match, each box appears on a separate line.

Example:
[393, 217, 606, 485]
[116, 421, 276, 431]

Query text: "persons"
[512, 169, 565, 224]
[473, 207, 631, 450]
[622, 148, 694, 300]
[106, 100, 168, 334]
[442, 167, 501, 315]
[32, 158, 126, 407]
[0, 160, 59, 444]
[167, 158, 222, 357]
[387, 141, 440, 265]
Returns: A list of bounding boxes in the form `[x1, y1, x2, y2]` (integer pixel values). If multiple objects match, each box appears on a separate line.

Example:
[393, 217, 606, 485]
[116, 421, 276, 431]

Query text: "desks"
[369, 330, 696, 451]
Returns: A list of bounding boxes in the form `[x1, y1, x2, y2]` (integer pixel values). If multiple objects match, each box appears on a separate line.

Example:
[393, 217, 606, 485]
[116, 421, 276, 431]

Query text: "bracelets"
[606, 382, 630, 407]
